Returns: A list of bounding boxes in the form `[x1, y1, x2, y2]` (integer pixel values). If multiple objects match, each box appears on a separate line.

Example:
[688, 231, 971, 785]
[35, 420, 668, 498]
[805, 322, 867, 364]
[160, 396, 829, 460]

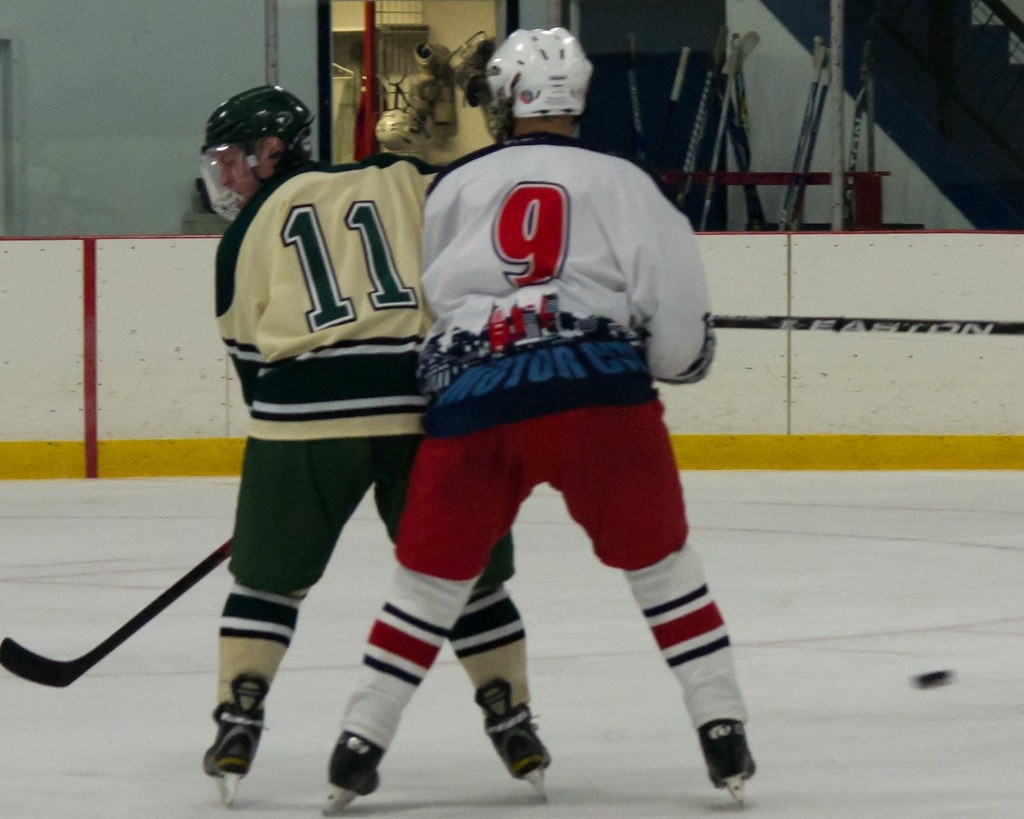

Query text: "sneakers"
[474, 676, 553, 804]
[202, 672, 270, 808]
[325, 730, 388, 815]
[696, 719, 756, 809]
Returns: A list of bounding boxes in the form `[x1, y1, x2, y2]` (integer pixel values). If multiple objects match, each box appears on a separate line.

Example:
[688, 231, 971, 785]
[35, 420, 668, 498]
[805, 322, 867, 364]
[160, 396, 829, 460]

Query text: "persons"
[316, 26, 762, 817]
[199, 83, 552, 810]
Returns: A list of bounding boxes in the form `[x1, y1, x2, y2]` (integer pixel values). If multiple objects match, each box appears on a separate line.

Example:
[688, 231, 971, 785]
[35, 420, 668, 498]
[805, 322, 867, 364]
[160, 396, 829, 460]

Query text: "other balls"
[919, 671, 950, 687]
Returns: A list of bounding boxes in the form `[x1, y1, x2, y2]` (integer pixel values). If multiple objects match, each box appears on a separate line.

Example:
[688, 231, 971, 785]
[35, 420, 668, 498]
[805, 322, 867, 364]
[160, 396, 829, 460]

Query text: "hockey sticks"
[629, 32, 877, 231]
[1, 537, 231, 688]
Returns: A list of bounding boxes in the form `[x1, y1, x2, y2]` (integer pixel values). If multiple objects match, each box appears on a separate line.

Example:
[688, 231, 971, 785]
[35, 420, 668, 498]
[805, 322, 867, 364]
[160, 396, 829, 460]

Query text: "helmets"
[481, 27, 593, 144]
[375, 108, 431, 152]
[202, 86, 315, 222]
